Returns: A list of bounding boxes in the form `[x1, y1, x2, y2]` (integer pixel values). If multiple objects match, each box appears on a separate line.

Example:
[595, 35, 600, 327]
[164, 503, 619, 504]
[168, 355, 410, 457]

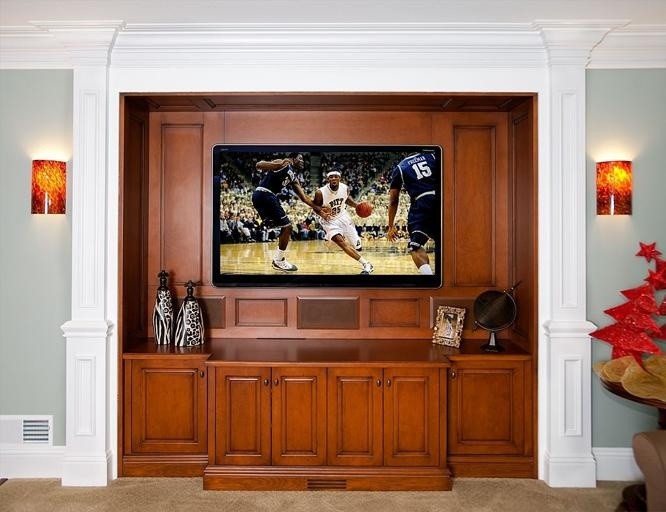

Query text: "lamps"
[30, 159, 65, 215]
[596, 162, 632, 215]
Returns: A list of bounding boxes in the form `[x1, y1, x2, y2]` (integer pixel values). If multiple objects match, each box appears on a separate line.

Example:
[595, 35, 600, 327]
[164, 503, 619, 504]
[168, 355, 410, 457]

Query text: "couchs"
[634, 430, 665, 512]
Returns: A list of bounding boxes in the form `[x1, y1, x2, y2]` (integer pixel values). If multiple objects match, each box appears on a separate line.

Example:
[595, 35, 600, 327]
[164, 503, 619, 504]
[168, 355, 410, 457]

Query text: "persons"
[220, 151, 436, 246]
[312, 167, 376, 274]
[251, 152, 334, 272]
[389, 152, 438, 276]
[442, 316, 457, 337]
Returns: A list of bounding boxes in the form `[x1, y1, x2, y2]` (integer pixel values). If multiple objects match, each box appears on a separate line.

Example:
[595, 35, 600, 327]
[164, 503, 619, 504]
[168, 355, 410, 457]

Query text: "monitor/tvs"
[210, 144, 444, 287]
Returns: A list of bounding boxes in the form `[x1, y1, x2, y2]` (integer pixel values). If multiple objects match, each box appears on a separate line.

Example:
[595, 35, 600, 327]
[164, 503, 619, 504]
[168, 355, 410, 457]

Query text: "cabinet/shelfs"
[214, 365, 327, 466]
[327, 367, 440, 466]
[130, 359, 207, 455]
[447, 362, 525, 455]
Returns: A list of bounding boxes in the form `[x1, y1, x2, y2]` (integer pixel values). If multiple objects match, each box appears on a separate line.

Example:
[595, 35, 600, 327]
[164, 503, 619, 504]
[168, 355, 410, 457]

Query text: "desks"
[600, 370, 666, 512]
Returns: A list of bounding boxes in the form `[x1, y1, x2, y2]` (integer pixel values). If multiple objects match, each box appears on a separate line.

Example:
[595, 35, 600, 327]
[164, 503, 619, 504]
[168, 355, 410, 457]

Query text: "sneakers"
[355, 238, 362, 251]
[273, 257, 298, 272]
[361, 262, 374, 275]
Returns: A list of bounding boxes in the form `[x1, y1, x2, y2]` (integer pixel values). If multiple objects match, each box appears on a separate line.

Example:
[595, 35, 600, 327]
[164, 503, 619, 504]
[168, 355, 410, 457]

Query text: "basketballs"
[357, 202, 372, 217]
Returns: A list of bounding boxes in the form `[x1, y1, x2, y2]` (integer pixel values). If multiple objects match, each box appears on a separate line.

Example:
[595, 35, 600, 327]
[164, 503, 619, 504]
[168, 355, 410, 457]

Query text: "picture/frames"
[432, 305, 467, 349]
